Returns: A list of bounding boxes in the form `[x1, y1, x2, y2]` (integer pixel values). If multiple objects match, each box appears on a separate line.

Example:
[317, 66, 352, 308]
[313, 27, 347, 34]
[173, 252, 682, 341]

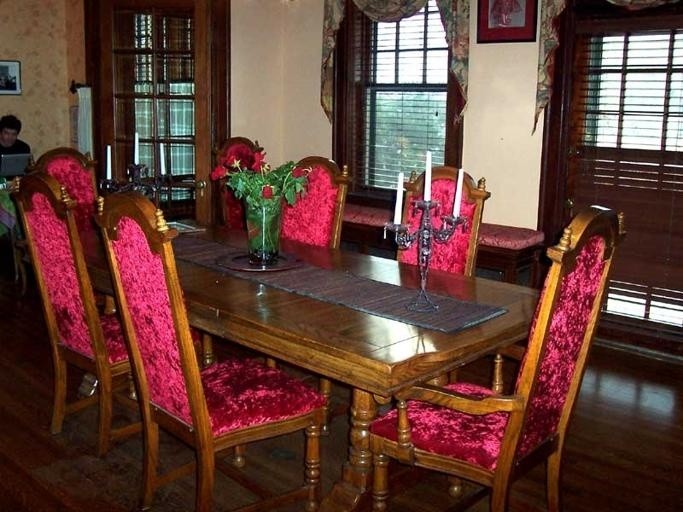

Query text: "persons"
[0, 114, 31, 184]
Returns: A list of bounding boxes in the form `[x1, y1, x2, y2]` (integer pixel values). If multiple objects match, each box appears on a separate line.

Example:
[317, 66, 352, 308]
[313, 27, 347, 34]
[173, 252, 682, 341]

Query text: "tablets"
[0, 153, 33, 177]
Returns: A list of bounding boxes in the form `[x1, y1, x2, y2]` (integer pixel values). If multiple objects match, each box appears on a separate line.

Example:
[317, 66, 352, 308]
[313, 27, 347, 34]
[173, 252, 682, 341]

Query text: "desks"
[65, 222, 546, 507]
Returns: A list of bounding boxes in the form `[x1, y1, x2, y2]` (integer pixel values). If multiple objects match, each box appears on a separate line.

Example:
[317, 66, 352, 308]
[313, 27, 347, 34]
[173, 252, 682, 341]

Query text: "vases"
[241, 203, 283, 264]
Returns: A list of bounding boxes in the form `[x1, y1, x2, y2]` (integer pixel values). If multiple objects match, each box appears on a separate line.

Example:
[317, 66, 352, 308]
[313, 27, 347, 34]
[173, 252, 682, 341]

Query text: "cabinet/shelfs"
[101, 0, 227, 222]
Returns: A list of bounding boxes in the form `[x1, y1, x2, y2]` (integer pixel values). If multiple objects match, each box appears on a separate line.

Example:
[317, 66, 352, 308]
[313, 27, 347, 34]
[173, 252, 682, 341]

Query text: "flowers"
[208, 146, 313, 248]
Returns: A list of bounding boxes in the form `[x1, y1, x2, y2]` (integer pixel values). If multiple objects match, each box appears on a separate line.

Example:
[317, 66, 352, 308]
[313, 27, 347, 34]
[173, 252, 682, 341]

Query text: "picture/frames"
[476, 0, 538, 45]
[0, 59, 23, 97]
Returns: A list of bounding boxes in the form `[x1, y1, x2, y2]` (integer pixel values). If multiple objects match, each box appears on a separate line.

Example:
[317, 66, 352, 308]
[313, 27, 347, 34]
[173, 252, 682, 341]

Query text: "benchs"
[340, 199, 548, 288]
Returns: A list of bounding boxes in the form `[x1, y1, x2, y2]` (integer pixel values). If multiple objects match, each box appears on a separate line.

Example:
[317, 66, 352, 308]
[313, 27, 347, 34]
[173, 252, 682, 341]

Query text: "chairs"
[84, 192, 329, 509]
[245, 156, 357, 435]
[367, 205, 622, 509]
[11, 170, 146, 454]
[381, 166, 488, 407]
[27, 145, 154, 405]
[186, 135, 261, 365]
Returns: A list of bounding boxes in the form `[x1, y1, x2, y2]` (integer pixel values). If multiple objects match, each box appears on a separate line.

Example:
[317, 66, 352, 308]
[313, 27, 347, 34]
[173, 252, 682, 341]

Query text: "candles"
[453, 167, 464, 217]
[159, 141, 167, 175]
[133, 131, 140, 166]
[105, 145, 113, 181]
[425, 149, 431, 201]
[392, 170, 404, 225]
